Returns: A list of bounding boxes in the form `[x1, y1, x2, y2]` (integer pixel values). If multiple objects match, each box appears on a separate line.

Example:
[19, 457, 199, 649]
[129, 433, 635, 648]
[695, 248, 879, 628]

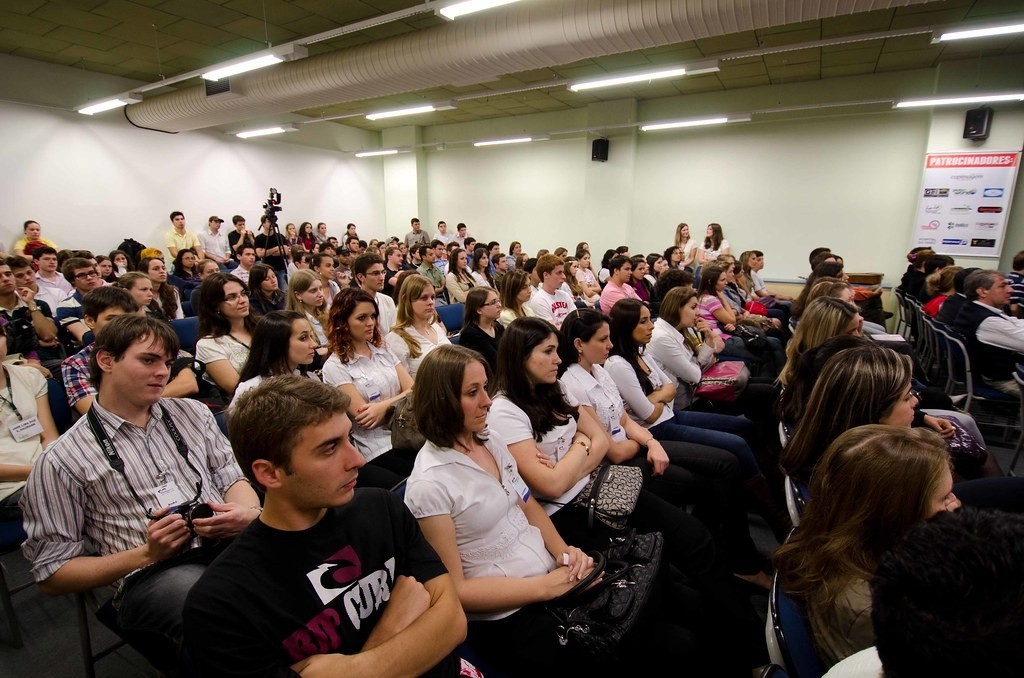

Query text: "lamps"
[235, 122, 299, 139]
[434, 0, 521, 21]
[73, 91, 143, 116]
[365, 100, 459, 120]
[930, 17, 1024, 44]
[891, 90, 1024, 110]
[640, 113, 752, 132]
[472, 133, 551, 146]
[200, 43, 308, 82]
[352, 149, 400, 158]
[567, 58, 720, 92]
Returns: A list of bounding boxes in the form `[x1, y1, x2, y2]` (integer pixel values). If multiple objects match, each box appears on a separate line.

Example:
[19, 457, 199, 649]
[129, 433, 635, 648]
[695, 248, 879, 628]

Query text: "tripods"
[258, 214, 289, 275]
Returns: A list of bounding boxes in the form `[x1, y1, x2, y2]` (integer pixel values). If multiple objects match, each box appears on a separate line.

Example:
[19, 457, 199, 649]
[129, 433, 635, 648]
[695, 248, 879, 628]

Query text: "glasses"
[75, 272, 98, 279]
[222, 291, 251, 301]
[483, 299, 501, 305]
[366, 270, 386, 276]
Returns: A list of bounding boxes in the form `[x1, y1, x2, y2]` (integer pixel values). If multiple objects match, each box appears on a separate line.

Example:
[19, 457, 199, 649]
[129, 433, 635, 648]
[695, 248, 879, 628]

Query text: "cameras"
[162, 500, 214, 543]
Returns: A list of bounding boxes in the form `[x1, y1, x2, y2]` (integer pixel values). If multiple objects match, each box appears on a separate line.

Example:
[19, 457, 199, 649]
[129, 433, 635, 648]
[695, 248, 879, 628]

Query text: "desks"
[844, 273, 885, 285]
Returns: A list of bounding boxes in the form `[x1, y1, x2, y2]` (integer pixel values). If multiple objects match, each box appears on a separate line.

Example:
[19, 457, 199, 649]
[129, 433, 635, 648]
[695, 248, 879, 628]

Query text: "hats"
[336, 246, 352, 255]
[209, 216, 224, 222]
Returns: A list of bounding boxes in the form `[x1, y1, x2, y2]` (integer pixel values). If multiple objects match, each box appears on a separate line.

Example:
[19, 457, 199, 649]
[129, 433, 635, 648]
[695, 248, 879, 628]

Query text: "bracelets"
[644, 437, 656, 448]
[572, 439, 591, 456]
[250, 505, 263, 512]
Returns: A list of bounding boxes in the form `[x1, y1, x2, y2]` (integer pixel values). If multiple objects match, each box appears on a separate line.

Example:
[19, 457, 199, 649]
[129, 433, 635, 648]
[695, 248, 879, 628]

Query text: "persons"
[0, 211, 1024, 597]
[181, 373, 492, 678]
[820, 506, 1024, 678]
[403, 344, 708, 678]
[770, 421, 964, 671]
[18, 313, 261, 678]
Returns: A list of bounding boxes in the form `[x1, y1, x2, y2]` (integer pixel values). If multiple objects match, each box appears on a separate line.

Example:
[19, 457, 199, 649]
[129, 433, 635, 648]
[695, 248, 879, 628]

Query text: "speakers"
[592, 139, 609, 162]
[962, 108, 994, 141]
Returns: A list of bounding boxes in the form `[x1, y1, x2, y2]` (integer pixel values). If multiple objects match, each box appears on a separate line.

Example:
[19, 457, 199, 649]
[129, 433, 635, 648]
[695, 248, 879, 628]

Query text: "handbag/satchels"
[388, 392, 426, 448]
[758, 294, 777, 308]
[744, 301, 767, 316]
[544, 528, 664, 667]
[924, 415, 988, 472]
[737, 318, 768, 332]
[717, 321, 769, 353]
[689, 361, 751, 409]
[572, 463, 644, 530]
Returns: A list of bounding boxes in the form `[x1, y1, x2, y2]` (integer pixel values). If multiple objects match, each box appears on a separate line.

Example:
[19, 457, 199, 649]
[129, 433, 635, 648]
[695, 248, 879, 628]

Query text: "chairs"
[1, 285, 1024, 678]
